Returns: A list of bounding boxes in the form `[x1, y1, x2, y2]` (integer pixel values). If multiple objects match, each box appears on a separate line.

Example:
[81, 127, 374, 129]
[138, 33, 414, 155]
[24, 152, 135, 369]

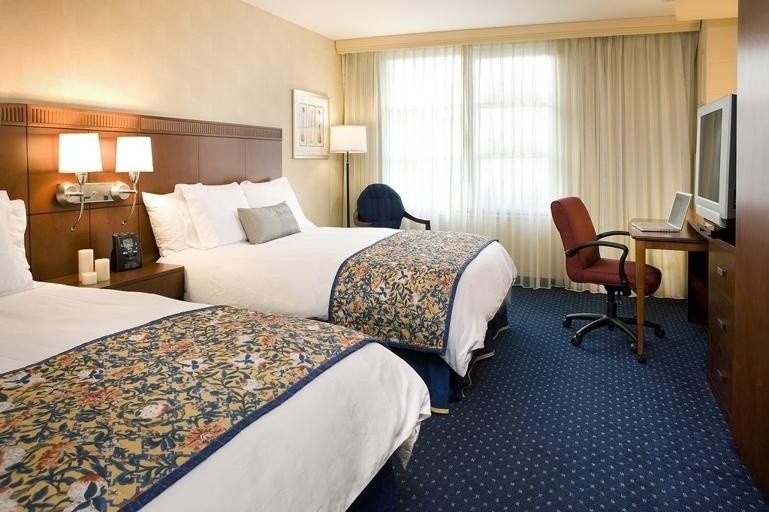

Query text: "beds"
[157, 228, 510, 413]
[0, 280, 393, 512]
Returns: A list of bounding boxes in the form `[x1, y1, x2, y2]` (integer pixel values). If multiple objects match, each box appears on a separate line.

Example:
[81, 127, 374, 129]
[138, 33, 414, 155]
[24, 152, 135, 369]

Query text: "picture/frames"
[292, 89, 331, 160]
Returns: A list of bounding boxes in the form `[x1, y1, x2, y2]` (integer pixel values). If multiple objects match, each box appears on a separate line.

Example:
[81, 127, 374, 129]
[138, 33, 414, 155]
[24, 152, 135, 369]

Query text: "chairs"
[551, 197, 664, 353]
[353, 183, 430, 232]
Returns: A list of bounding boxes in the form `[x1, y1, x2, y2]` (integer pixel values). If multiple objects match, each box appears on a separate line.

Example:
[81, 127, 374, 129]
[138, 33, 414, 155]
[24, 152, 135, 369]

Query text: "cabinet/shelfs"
[45, 263, 186, 301]
[704, 239, 737, 433]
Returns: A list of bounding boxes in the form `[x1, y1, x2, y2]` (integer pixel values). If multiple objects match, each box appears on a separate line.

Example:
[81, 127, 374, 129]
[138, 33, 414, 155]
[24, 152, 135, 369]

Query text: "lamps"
[330, 125, 366, 227]
[59, 132, 102, 231]
[115, 136, 153, 225]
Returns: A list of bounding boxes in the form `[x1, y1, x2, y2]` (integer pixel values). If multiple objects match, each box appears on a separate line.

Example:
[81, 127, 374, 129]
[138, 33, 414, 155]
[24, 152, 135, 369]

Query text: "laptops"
[631, 191, 694, 232]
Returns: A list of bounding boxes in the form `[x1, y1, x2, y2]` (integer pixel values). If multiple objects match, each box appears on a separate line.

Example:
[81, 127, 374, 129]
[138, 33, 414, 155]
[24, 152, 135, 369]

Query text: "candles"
[82, 273, 98, 285]
[95, 259, 110, 281]
[78, 250, 93, 280]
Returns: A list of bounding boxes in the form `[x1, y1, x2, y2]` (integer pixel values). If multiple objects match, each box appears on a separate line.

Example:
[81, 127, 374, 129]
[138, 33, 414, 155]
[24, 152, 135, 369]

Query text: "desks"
[628, 218, 709, 365]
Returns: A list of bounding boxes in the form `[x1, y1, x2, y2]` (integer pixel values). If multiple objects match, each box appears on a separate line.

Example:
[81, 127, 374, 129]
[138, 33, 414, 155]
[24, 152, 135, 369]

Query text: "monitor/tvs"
[693, 92, 737, 229]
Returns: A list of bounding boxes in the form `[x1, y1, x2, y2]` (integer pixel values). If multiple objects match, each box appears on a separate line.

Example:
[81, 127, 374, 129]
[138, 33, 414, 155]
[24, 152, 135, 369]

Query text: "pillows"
[238, 200, 301, 245]
[0, 199, 34, 297]
[175, 182, 251, 251]
[240, 177, 317, 229]
[142, 182, 203, 257]
